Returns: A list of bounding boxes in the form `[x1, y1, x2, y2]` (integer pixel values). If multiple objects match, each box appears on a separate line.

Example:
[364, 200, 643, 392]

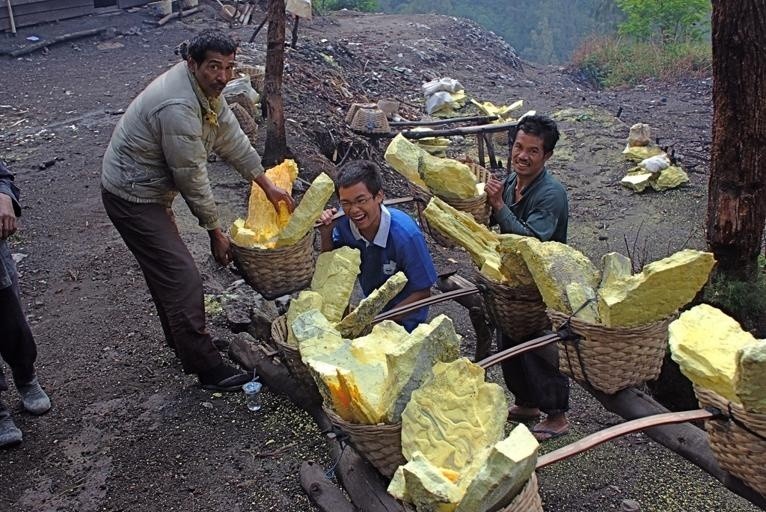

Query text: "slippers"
[529, 420, 570, 440]
[505, 410, 541, 420]
[202, 369, 260, 391]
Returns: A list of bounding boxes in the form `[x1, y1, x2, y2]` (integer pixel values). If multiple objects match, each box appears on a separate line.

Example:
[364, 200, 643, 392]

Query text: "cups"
[242, 381, 263, 411]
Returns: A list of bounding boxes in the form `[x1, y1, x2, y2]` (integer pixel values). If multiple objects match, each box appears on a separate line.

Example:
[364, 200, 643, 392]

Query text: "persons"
[314, 159, 436, 335]
[0, 158, 52, 447]
[454, 113, 569, 442]
[99, 30, 296, 393]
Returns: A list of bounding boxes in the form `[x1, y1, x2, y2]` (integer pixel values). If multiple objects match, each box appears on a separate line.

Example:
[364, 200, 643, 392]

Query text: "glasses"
[338, 197, 373, 209]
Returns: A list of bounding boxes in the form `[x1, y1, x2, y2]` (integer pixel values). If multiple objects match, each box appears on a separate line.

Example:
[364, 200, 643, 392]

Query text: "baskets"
[345, 103, 391, 134]
[271, 304, 371, 384]
[322, 398, 406, 479]
[401, 469, 544, 512]
[220, 5, 240, 22]
[469, 256, 679, 395]
[408, 163, 493, 248]
[223, 61, 265, 136]
[692, 383, 765, 498]
[226, 224, 314, 302]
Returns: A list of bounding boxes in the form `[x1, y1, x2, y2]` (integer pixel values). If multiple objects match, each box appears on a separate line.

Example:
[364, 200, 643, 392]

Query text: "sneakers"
[0, 407, 23, 447]
[18, 376, 52, 415]
